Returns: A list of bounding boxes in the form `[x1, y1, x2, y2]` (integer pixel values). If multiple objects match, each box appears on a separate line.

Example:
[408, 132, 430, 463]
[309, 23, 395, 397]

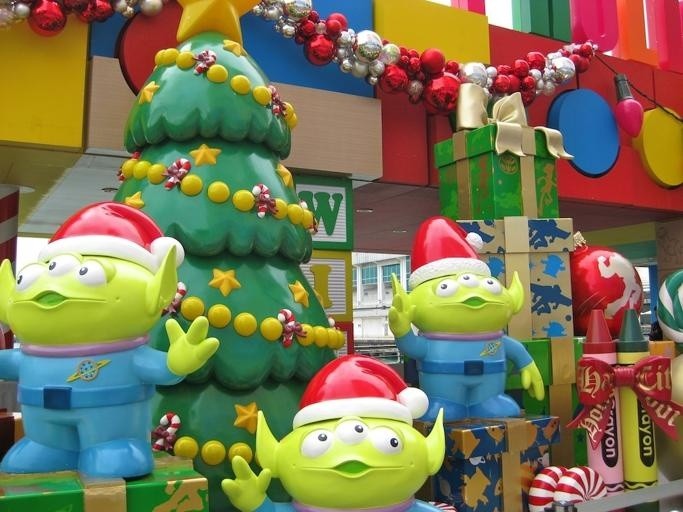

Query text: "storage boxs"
[433, 84, 575, 220]
[455, 216, 574, 341]
[427, 414, 561, 512]
[0, 448, 209, 512]
[505, 337, 588, 469]
